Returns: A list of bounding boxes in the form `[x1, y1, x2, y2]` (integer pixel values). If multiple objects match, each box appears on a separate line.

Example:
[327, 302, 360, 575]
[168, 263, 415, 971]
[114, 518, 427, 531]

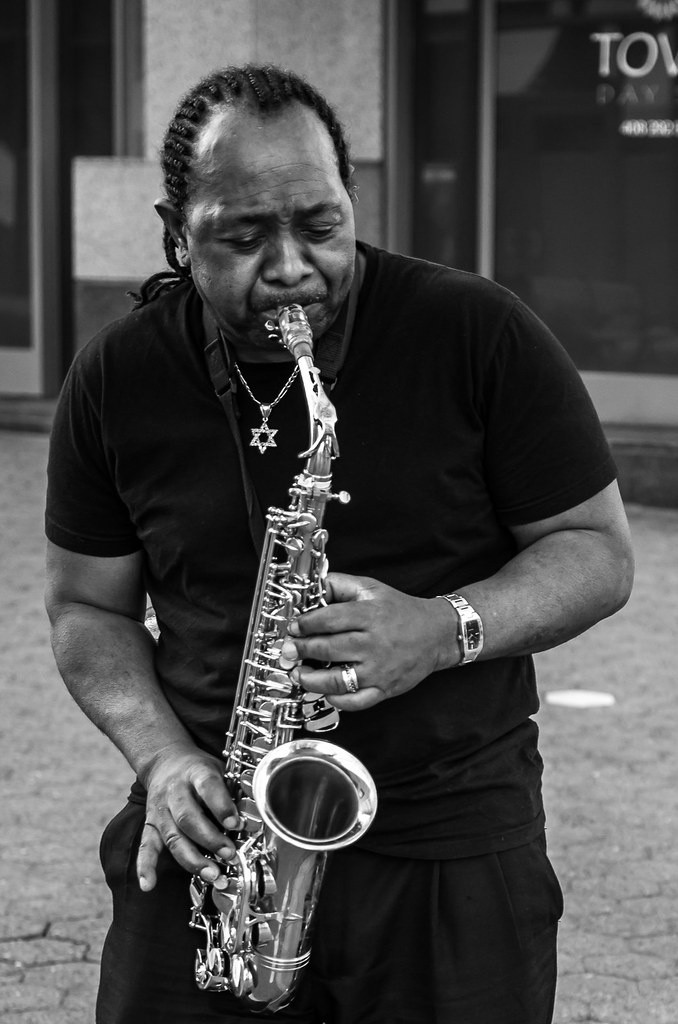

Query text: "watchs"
[434, 593, 484, 668]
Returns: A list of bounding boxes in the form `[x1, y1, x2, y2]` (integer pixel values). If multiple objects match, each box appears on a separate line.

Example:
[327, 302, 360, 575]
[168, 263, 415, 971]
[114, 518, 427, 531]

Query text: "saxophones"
[187, 302, 381, 1019]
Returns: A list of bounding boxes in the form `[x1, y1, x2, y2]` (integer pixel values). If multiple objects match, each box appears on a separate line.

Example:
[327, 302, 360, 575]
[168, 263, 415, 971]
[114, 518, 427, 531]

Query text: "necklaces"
[231, 356, 299, 456]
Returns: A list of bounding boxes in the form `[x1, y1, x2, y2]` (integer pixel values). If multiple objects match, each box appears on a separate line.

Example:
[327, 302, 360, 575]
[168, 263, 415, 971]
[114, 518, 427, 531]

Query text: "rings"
[342, 663, 360, 694]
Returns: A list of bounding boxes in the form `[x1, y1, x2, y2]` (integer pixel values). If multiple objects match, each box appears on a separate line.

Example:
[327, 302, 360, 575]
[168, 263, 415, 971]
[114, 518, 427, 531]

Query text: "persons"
[42, 60, 635, 1024]
